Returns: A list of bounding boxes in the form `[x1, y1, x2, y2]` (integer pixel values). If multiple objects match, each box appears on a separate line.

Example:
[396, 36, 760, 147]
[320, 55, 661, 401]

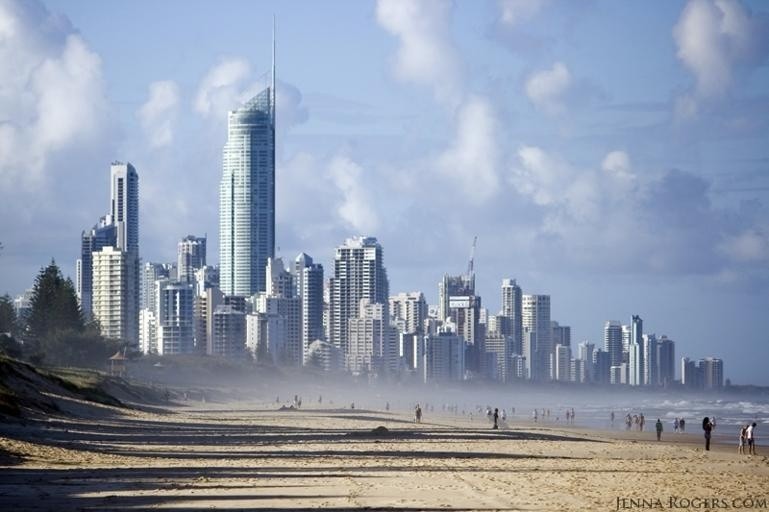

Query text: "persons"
[679, 417, 685, 433]
[672, 416, 679, 433]
[743, 421, 758, 455]
[702, 415, 717, 450]
[655, 417, 665, 441]
[624, 411, 646, 431]
[737, 424, 747, 455]
[273, 395, 576, 430]
